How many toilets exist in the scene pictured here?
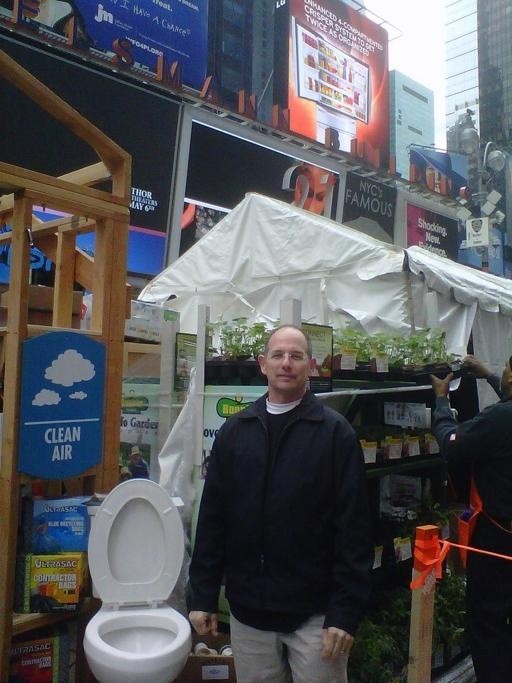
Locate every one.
[84,477,194,682]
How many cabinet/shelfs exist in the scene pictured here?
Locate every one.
[2,52,133,680]
[79,194,480,680]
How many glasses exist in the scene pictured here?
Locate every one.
[266,350,309,361]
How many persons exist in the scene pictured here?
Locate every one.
[188,324,376,682]
[118,445,150,484]
[292,162,336,219]
[424,345,511,682]
